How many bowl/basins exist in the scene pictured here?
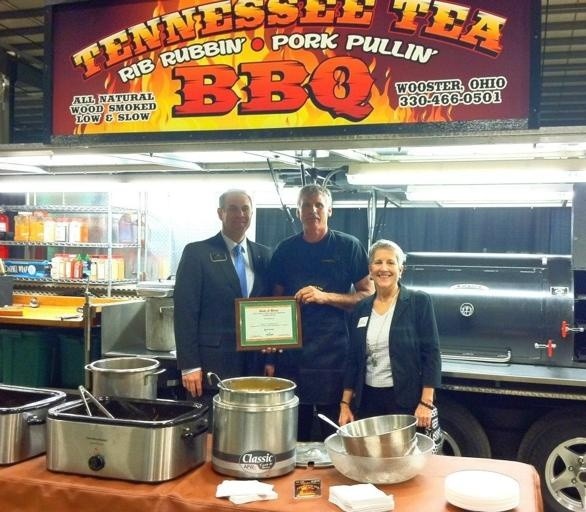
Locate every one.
[336,414,418,457]
[323,433,435,485]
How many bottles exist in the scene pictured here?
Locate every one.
[49,253,124,280]
[0,209,144,244]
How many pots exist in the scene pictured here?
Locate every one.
[83,358,166,401]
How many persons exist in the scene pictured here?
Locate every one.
[261,185,375,443]
[173,191,273,433]
[339,239,442,434]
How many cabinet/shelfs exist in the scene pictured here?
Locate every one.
[0,205,149,289]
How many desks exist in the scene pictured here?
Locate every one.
[0,434,542,512]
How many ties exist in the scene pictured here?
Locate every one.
[235,246,248,298]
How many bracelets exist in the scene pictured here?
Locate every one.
[339,399,351,406]
[419,400,434,412]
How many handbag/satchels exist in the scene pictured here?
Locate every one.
[421,404,444,454]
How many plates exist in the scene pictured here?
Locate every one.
[442,469,520,511]
